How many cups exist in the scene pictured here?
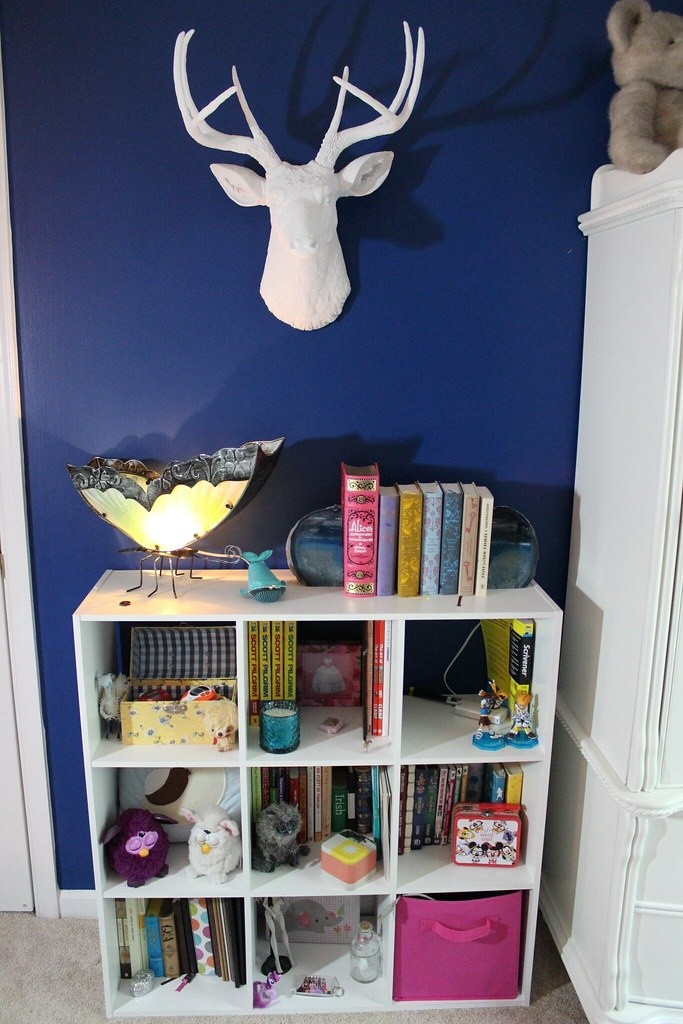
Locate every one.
[258,699,300,754]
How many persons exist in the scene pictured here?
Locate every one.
[262,896,295,975]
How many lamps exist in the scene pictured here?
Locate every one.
[64,436,288,601]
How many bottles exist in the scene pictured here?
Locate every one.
[349,921,380,984]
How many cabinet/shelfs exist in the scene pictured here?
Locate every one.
[71,566,563,1017]
[537,148,683,1024]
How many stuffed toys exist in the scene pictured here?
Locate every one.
[255,801,311,872]
[97,669,132,744]
[182,806,243,886]
[101,806,179,886]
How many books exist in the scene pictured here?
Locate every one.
[477,618,534,718]
[252,768,381,861]
[338,462,488,598]
[112,896,247,990]
[400,764,524,854]
[363,620,389,740]
[248,621,298,728]
